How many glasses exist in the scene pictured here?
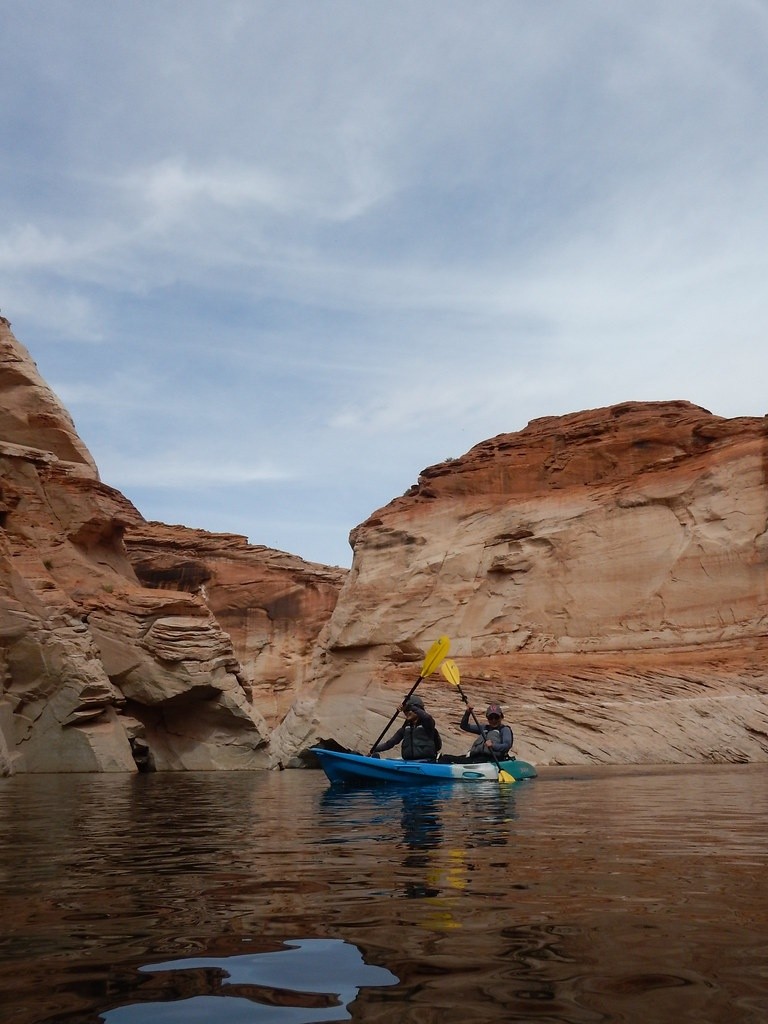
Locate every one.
[489,716,499,720]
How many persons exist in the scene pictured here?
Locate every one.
[441,701,514,764]
[366,696,442,763]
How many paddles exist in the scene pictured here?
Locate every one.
[367,635,450,756]
[440,659,517,782]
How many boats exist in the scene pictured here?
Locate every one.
[309,748,538,788]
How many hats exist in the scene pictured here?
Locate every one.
[405,695,426,711]
[486,705,502,718]
